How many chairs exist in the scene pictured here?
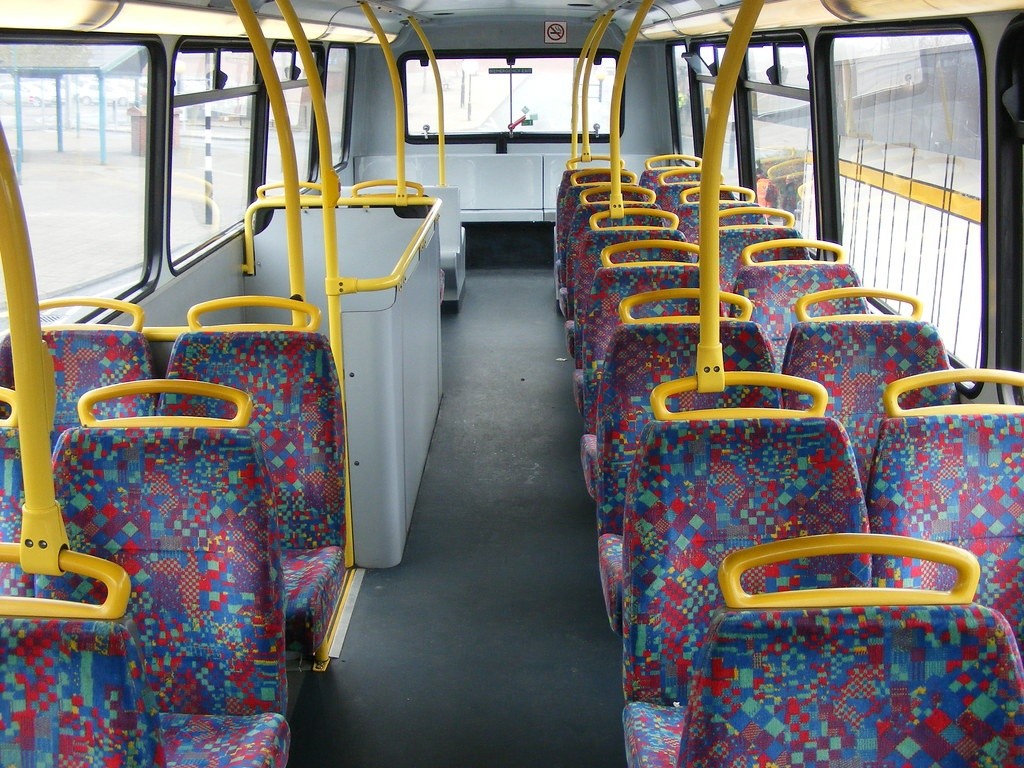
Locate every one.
[0,149,1022,764]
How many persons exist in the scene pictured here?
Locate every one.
[781,176,797,226]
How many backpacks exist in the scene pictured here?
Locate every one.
[765,182,777,202]
